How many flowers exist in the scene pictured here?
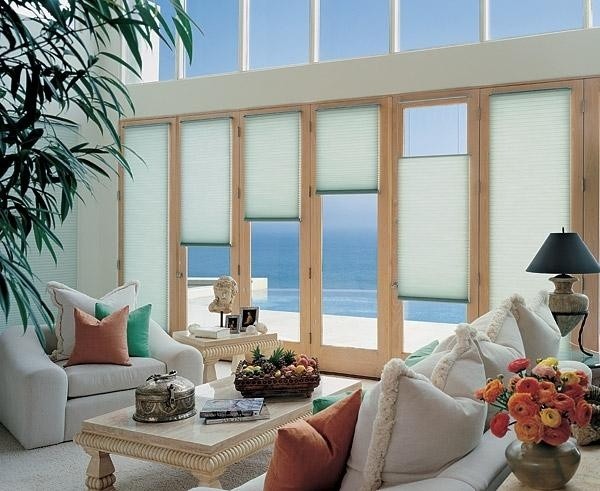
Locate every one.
[475,356,594,445]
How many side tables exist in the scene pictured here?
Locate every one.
[171,325,280,384]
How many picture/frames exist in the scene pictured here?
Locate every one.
[223,306,260,334]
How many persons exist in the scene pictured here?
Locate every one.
[228,318,236,330]
[242,311,253,327]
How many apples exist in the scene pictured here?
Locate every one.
[283,354,316,375]
[242,365,262,376]
[273,370,281,378]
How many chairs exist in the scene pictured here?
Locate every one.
[1,315,203,452]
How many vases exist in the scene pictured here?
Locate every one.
[502,431,582,490]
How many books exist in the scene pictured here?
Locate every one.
[204,403,271,425]
[199,396,265,418]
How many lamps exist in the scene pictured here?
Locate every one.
[521,229,599,358]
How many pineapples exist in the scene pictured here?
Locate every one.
[277,350,297,367]
[252,346,267,367]
[263,347,284,374]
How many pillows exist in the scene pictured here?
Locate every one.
[46,280,153,367]
